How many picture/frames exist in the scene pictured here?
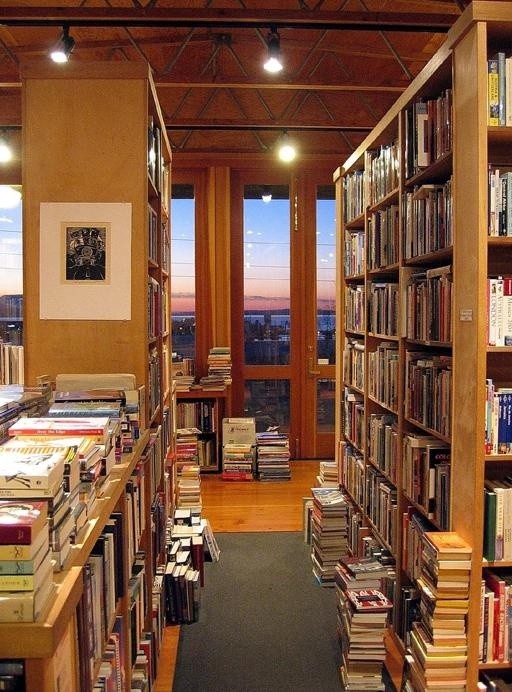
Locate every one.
[59,221,111,285]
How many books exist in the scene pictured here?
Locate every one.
[147,117,171,419]
[478,573,510,663]
[2,373,220,691]
[486,52,511,127]
[340,89,453,343]
[333,556,393,689]
[340,337,453,638]
[171,346,292,482]
[487,164,510,237]
[484,379,510,455]
[482,479,510,562]
[304,462,373,587]
[404,532,473,690]
[2,344,25,384]
[487,275,510,347]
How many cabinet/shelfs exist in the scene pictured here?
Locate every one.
[50,37,76,63]
[22,60,182,631]
[333,1,512,692]
[176,385,228,472]
[0,433,179,692]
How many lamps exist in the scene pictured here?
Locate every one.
[264,33,282,73]
